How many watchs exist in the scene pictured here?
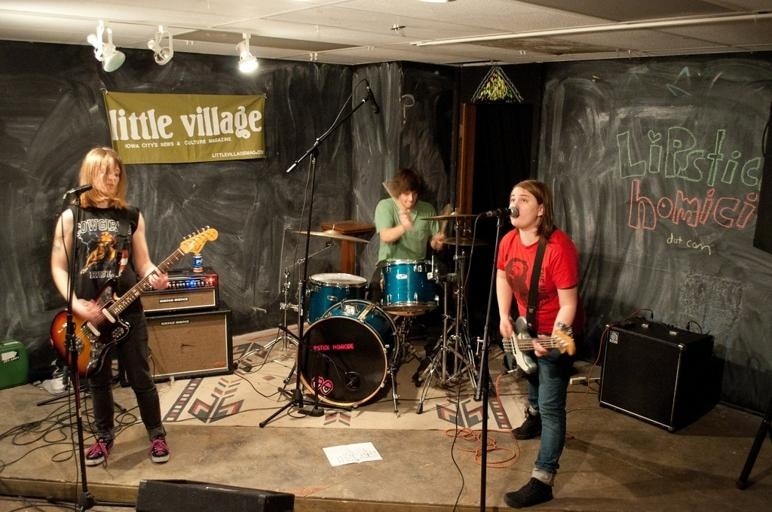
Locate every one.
[555,322,575,338]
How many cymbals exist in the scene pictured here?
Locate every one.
[419,208,476,220]
[291,225,372,244]
[444,237,487,247]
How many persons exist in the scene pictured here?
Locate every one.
[503,258,529,296]
[368,169,448,365]
[50,146,171,467]
[496,180,580,509]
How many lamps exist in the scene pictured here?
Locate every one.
[84,16,259,76]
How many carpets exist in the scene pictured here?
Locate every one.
[149,339,526,435]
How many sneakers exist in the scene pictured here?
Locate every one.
[149,435,170,462]
[512,407,543,440]
[84,436,114,466]
[504,477,554,509]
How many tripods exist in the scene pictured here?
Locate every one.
[414,222,496,414]
[233,243,335,373]
[258,96,366,429]
[47,194,136,512]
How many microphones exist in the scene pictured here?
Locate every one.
[487,208,519,217]
[366,83,380,114]
[69,185,92,195]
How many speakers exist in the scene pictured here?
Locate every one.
[600,317,713,432]
[118,300,234,388]
[137,479,294,512]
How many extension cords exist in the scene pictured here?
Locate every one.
[570,377,588,385]
[589,378,601,384]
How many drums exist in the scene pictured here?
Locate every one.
[298,300,399,407]
[379,258,439,315]
[306,273,369,326]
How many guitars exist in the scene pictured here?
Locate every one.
[51,225,219,377]
[500,317,575,379]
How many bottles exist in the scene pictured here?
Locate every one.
[191,253,204,273]
